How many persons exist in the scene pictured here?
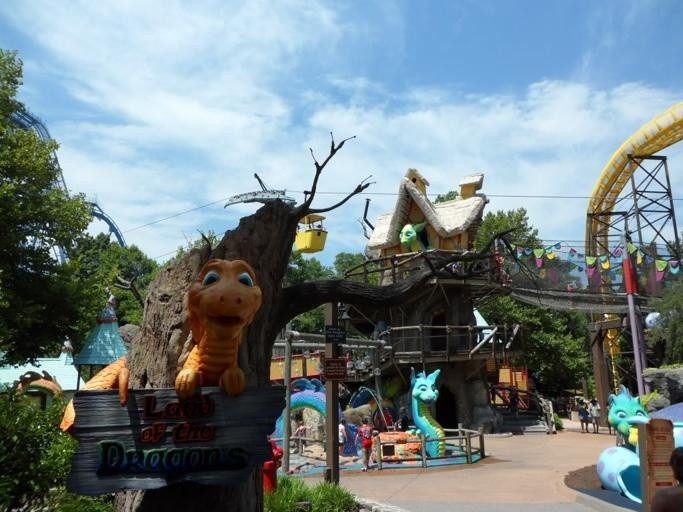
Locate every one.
[291,420,313,447]
[338,418,347,455]
[355,417,373,472]
[398,405,409,431]
[574,396,616,435]
